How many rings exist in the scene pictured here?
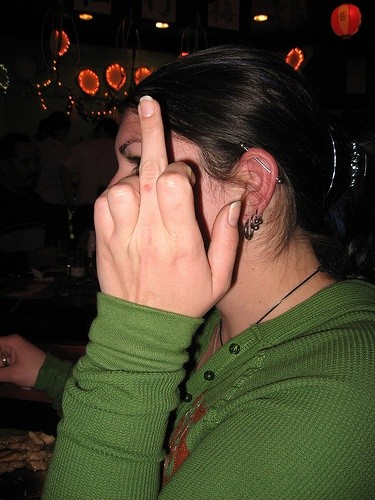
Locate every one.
[2,355,7,367]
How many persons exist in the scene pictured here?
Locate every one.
[1,133,63,278]
[0,45,375,500]
[63,119,118,249]
[34,109,75,239]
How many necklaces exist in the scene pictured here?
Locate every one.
[218,264,320,348]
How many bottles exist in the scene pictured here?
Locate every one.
[66,248,85,288]
